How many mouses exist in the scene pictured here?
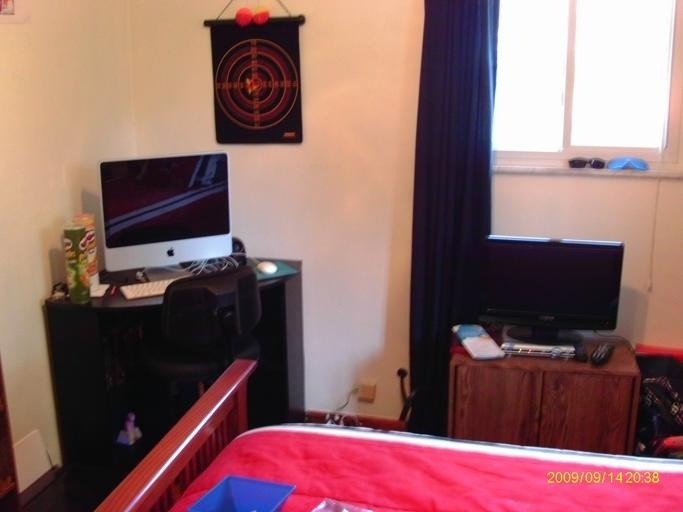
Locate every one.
[256,261,277,274]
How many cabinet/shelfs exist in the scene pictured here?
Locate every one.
[46,258,303,510]
[445,334,641,454]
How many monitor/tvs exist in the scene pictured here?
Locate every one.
[479,236,623,345]
[98,150,233,281]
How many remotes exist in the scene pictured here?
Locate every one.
[576,345,587,361]
[592,343,615,364]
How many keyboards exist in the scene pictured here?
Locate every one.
[120,278,181,300]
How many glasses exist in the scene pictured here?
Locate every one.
[567,157,605,169]
[606,156,649,171]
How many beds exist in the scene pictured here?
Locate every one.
[94,359,683,510]
